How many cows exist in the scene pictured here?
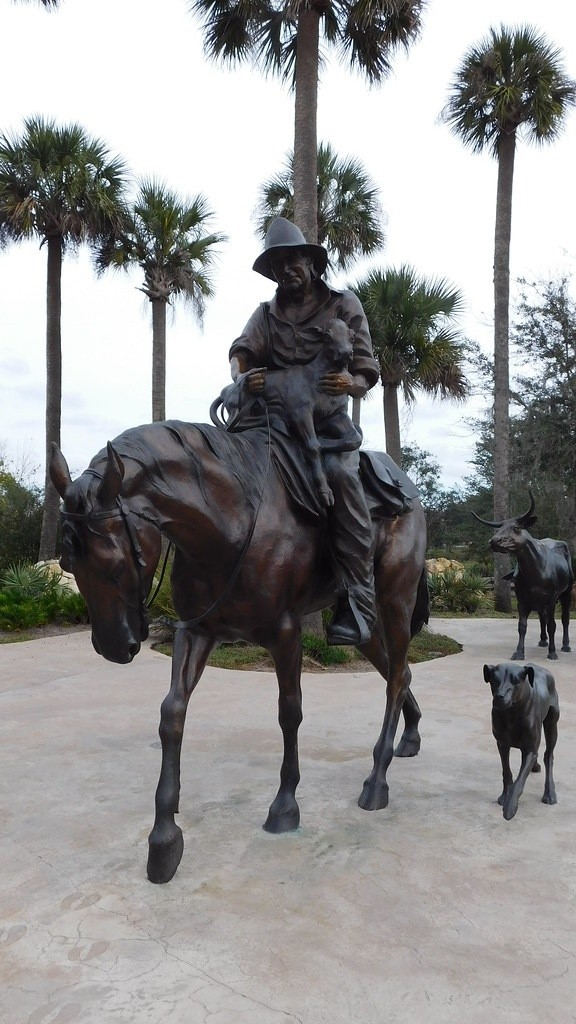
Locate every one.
[469,488,574,661]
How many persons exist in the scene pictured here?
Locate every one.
[227,215,380,646]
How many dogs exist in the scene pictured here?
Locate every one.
[483,661,558,820]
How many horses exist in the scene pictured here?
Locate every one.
[48,418,432,885]
